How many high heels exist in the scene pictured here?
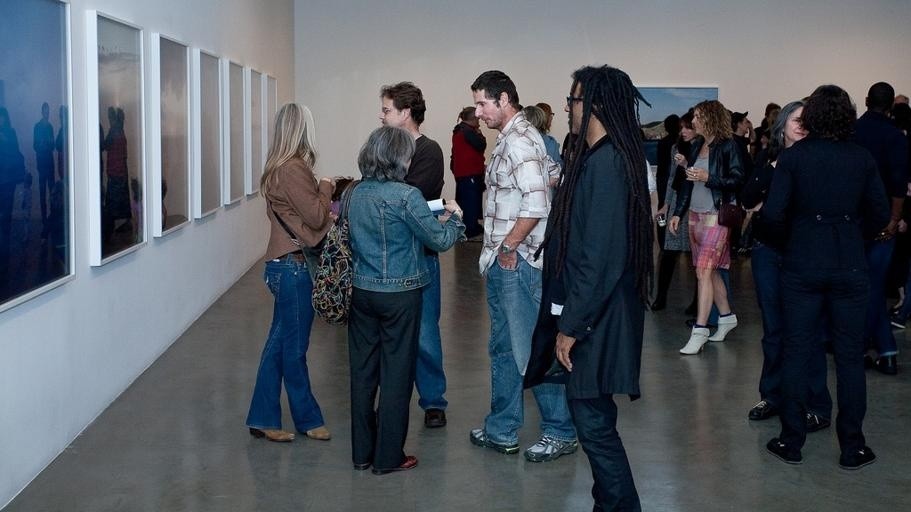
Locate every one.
[679,325,711,355]
[708,314,739,343]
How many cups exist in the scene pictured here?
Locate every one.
[689,167,698,170]
[657,215,667,226]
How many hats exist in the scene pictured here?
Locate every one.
[730,110,747,130]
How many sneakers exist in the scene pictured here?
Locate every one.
[748,400,777,420]
[839,448,874,470]
[807,413,831,432]
[766,438,802,462]
[523,435,577,463]
[466,234,484,243]
[874,353,898,375]
[891,306,908,330]
[471,429,520,454]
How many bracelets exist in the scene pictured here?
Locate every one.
[451,209,465,217]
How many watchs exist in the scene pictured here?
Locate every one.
[499,243,518,254]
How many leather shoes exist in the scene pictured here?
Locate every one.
[652,298,667,310]
[684,301,697,314]
[354,456,417,475]
[249,425,295,441]
[307,426,331,441]
[424,407,447,428]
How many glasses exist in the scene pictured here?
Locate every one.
[566,95,582,106]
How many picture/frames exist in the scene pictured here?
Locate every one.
[193,46,222,216]
[263,73,278,168]
[0,0,75,311]
[224,57,246,204]
[247,68,266,196]
[84,9,148,267]
[153,34,192,239]
[631,87,718,142]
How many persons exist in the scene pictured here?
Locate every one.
[469,70,579,462]
[645,83,910,470]
[533,64,655,512]
[1,78,168,266]
[378,81,448,429]
[340,127,467,473]
[451,104,574,242]
[331,176,354,217]
[246,105,336,443]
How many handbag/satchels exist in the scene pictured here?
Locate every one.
[299,245,321,281]
[717,202,745,230]
[312,219,354,328]
